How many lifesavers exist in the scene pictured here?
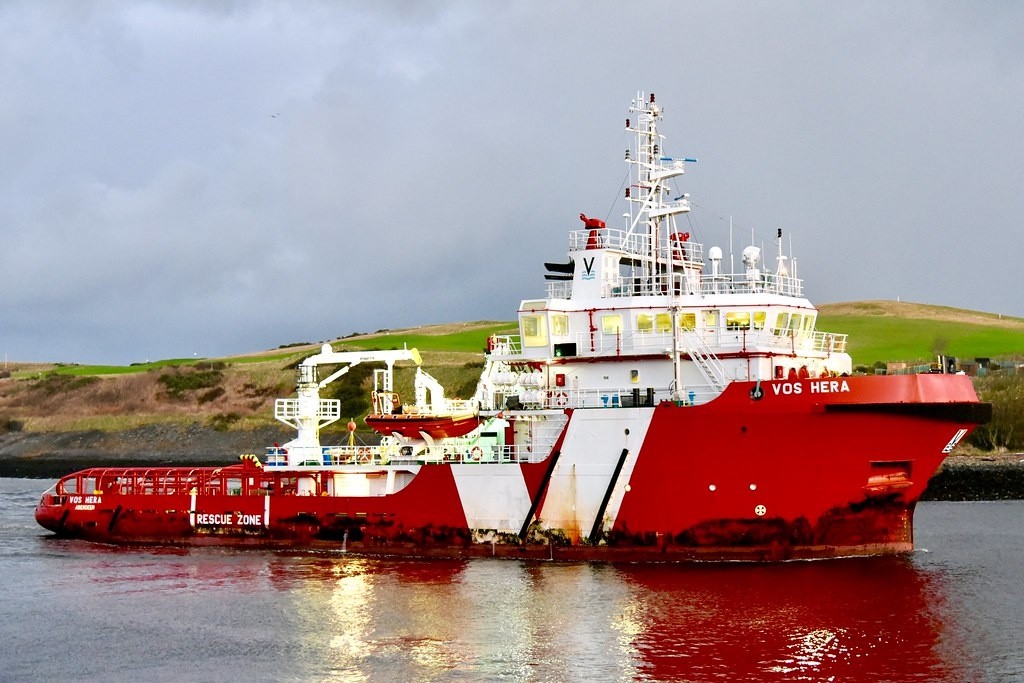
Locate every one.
[470,447,484,461]
[556,393,569,406]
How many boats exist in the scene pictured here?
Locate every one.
[34,93,992,559]
